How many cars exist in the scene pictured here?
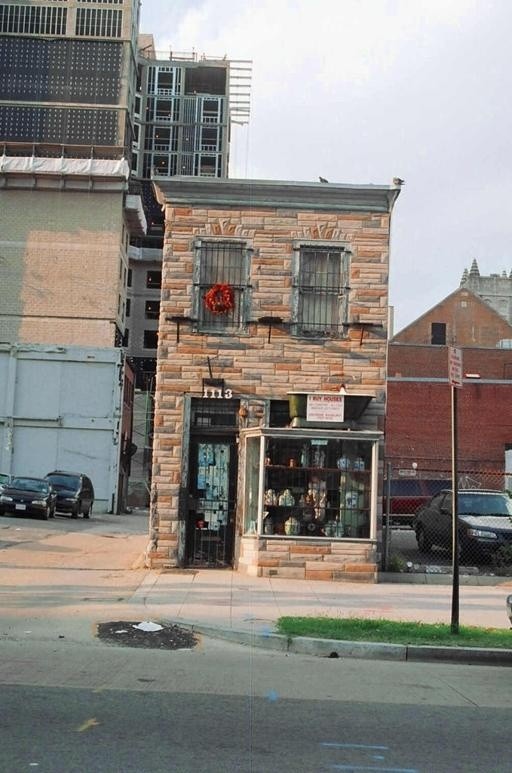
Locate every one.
[0,471,57,518]
[415,487,512,564]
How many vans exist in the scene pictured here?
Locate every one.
[384,477,453,526]
[43,469,95,520]
